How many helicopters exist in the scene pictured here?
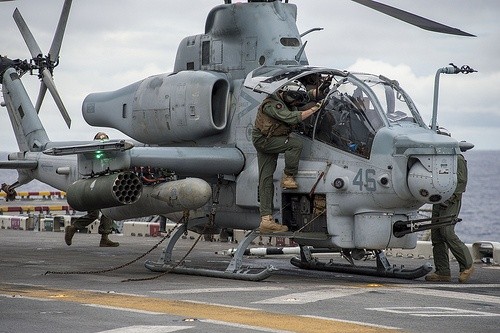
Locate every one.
[0,0,479,280]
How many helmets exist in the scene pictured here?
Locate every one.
[282,80,307,92]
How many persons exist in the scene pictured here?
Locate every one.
[425,154,473,283]
[65,209,119,247]
[252,82,324,234]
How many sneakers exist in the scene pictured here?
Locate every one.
[425,272,451,282]
[65,226,74,246]
[281,176,298,187]
[458,268,473,281]
[260,220,288,232]
[100,239,119,247]
